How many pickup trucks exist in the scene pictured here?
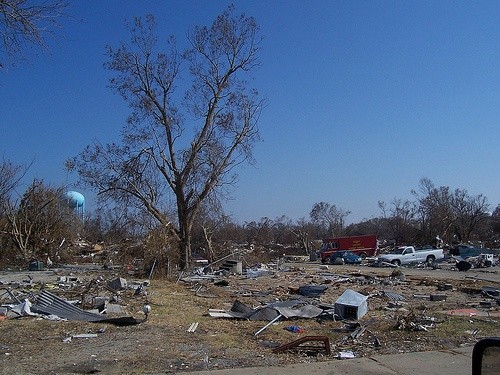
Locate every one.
[378,246,443,267]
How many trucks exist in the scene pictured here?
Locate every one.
[321,235,376,263]
